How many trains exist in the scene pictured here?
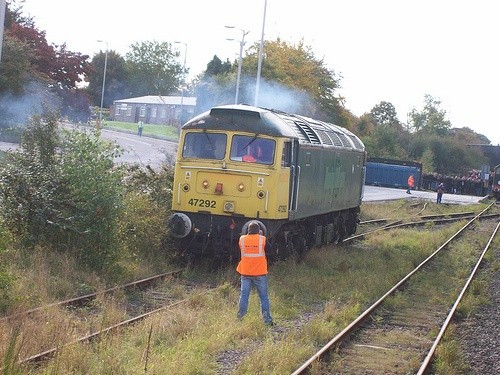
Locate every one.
[492,163,500,203]
[165,104,366,264]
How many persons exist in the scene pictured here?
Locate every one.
[406,169,495,204]
[137,119,144,137]
[236,221,277,326]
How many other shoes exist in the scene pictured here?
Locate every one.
[406,191,412,194]
[269,322,276,327]
